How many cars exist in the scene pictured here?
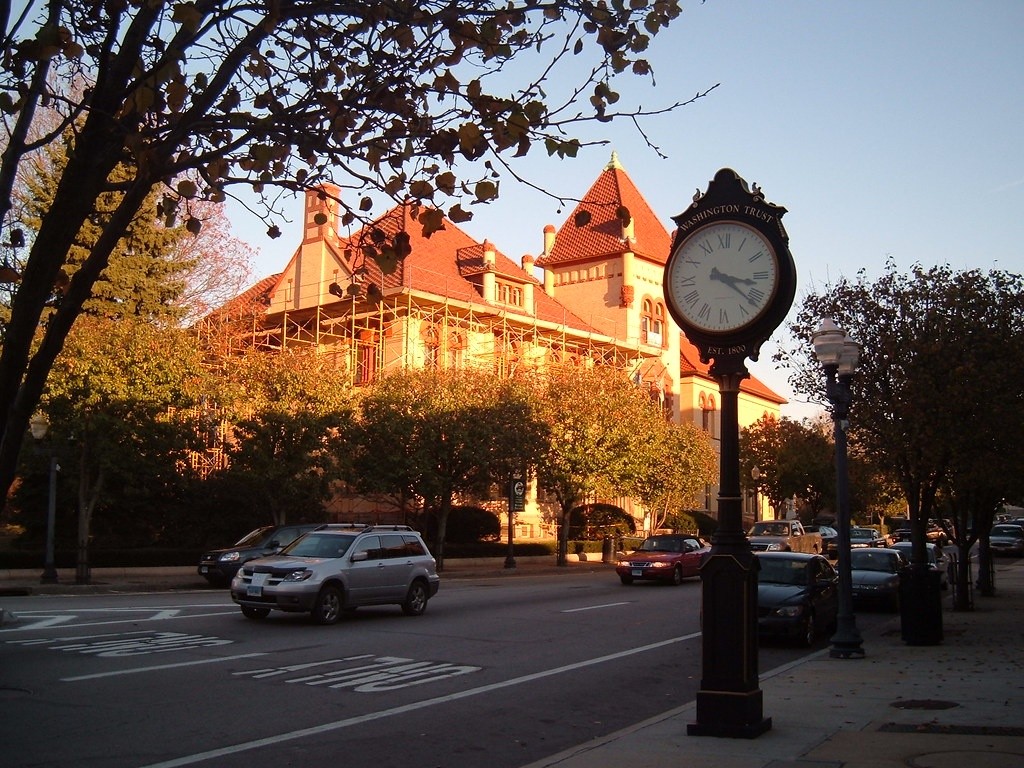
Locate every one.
[891,542,950,590]
[615,534,712,586]
[198,524,323,588]
[933,519,956,540]
[827,528,889,560]
[834,548,910,612]
[989,525,1024,556]
[893,519,947,545]
[699,552,839,648]
[792,525,838,554]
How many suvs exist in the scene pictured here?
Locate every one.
[231,523,440,625]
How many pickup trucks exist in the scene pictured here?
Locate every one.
[745,520,822,555]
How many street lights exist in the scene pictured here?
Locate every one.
[29,408,59,583]
[811,315,865,659]
[751,465,760,522]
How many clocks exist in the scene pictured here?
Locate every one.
[667,219,780,335]
